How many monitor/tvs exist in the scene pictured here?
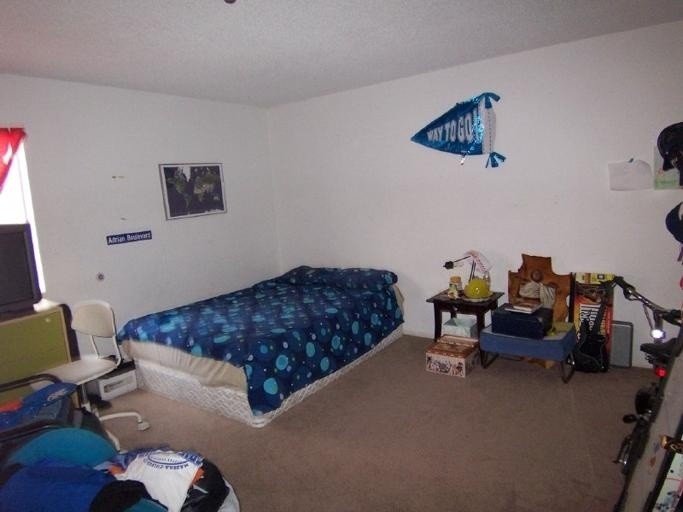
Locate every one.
[0,223,42,315]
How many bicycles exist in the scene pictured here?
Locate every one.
[611,274,683,511]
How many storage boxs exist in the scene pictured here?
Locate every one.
[85,361,138,399]
[443,317,477,337]
[492,302,554,340]
[425,335,480,378]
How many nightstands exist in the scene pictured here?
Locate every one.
[426,289,504,369]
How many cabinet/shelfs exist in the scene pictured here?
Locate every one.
[0,297,81,411]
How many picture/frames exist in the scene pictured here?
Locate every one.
[159,162,229,221]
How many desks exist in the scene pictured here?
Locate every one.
[479,324,577,384]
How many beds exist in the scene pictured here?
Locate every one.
[116,264,404,432]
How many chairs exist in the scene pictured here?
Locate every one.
[30,300,152,455]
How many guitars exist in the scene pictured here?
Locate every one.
[574,285,614,374]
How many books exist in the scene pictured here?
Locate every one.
[505,299,543,315]
